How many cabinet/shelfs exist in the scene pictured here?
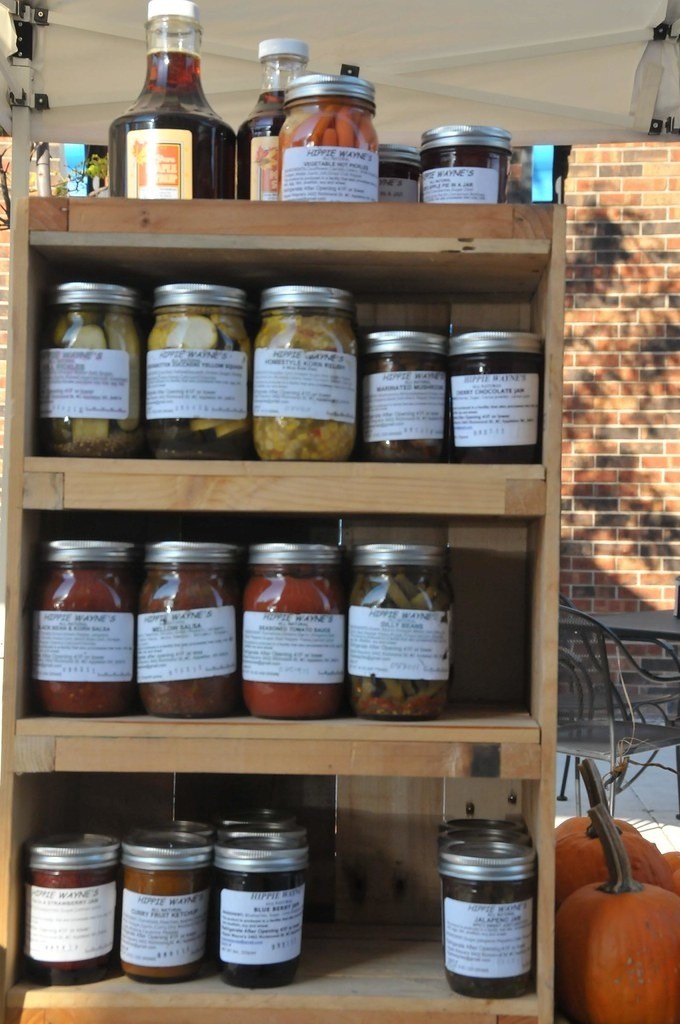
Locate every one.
[0,190,566,1024]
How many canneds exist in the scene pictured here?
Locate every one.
[437,818,536,1000]
[37,282,544,468]
[30,539,450,721]
[22,810,311,989]
[276,72,513,204]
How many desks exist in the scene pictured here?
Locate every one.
[560,609,680,671]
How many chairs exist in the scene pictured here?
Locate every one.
[557,603,680,818]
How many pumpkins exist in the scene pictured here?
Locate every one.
[553,758,680,1024]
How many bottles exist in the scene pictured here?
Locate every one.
[237,37,310,199]
[108,0,237,198]
